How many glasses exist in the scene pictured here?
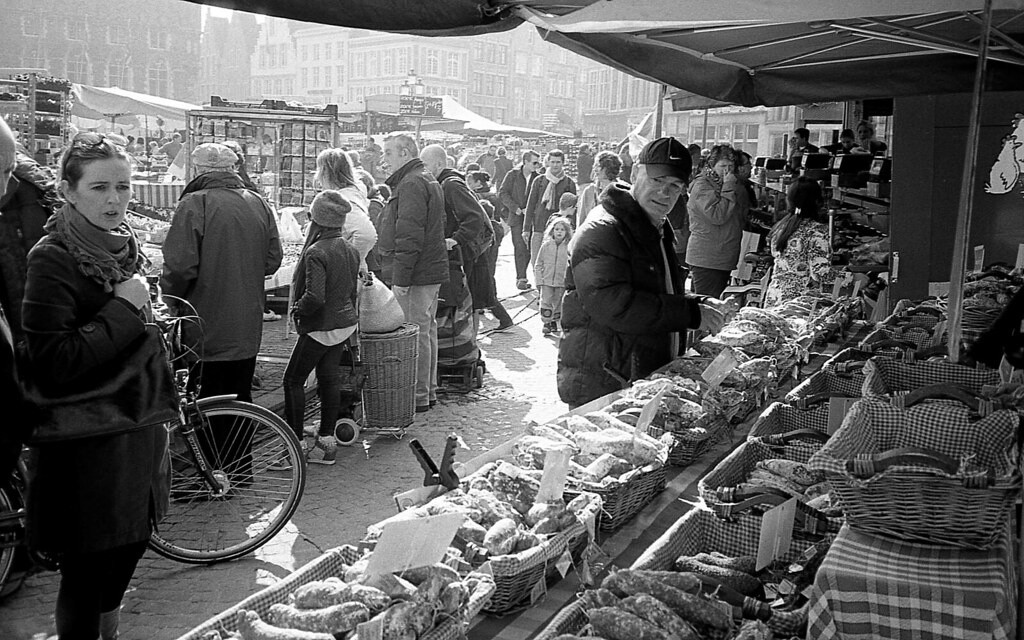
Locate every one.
[64,133,130,179]
[526,160,539,165]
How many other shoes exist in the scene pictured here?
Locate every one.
[306,440,337,465]
[543,324,552,337]
[516,280,531,289]
[550,321,558,333]
[262,310,281,320]
[493,319,514,333]
[173,483,235,501]
[267,452,294,471]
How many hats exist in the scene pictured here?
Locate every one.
[190,143,239,168]
[310,189,350,228]
[639,138,691,189]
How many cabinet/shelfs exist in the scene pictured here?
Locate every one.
[0,73,72,173]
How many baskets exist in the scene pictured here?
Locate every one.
[861,355,1024,420]
[809,398,1024,552]
[958,304,1004,332]
[181,296,863,640]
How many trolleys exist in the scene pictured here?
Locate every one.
[434,245,486,391]
[333,271,415,447]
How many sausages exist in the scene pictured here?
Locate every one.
[548,550,780,640]
[961,276,1009,309]
[209,413,659,640]
[738,458,837,511]
[605,294,840,431]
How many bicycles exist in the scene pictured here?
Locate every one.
[0,272,308,603]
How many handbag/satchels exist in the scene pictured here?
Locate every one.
[16,237,183,442]
[356,270,403,334]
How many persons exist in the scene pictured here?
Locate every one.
[0,119,888,577]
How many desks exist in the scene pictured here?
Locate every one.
[807,502,1021,640]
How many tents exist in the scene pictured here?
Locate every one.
[71,85,199,157]
